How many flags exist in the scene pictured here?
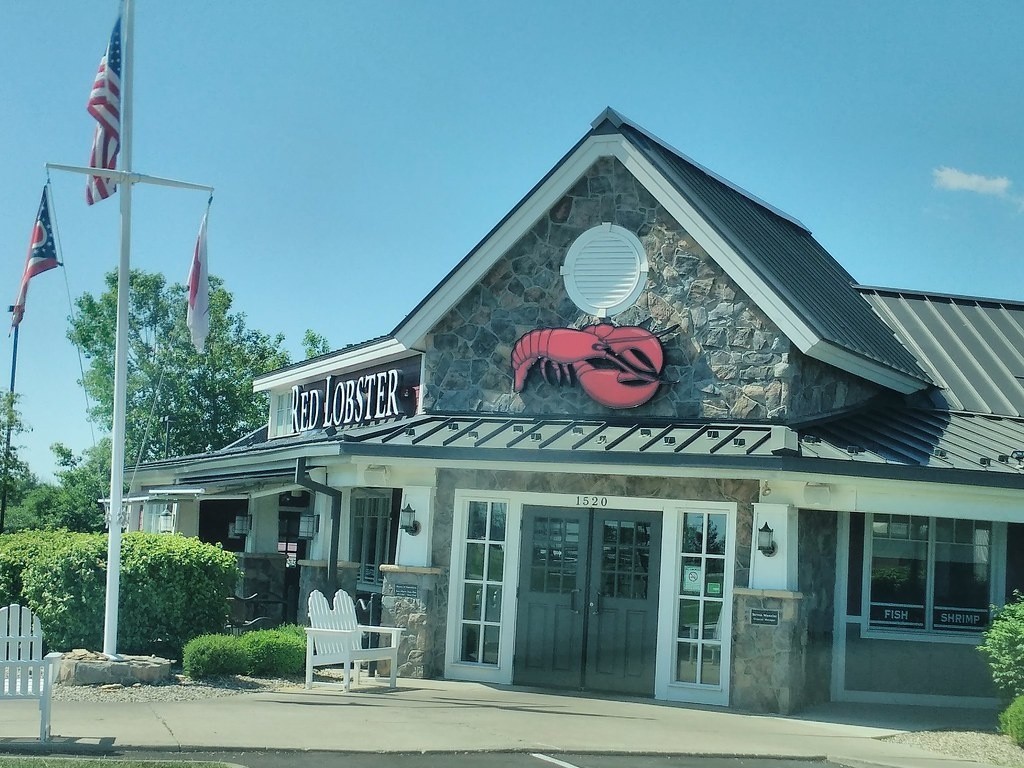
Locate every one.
[84,16,123,207]
[10,184,60,328]
[186,200,211,354]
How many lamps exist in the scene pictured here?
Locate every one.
[298,506,320,540]
[159,504,175,534]
[234,508,252,537]
[228,516,244,539]
[399,503,421,536]
[757,521,778,557]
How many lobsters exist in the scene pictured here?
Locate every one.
[510,317,681,409]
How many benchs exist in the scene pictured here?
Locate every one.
[303,588,408,694]
[1,604,63,742]
